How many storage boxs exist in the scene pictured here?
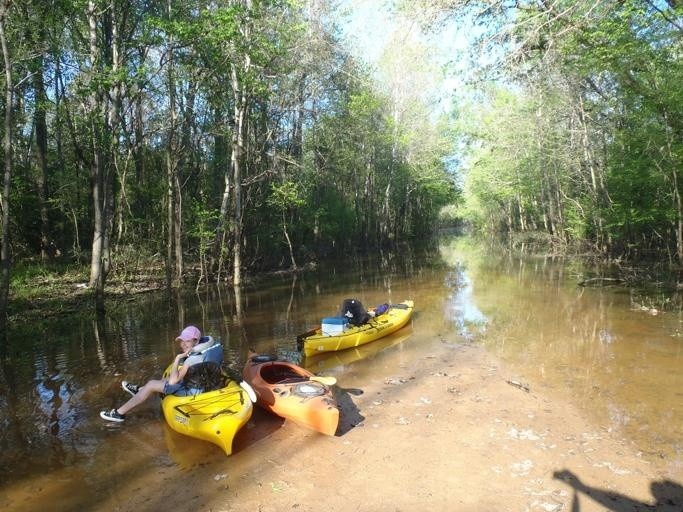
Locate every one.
[321,317,348,337]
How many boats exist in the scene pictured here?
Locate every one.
[244,349,341,435]
[296,300,414,357]
[162,361,254,456]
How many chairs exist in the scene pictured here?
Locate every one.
[184,361,222,394]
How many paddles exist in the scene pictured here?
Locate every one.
[275,372,336,386]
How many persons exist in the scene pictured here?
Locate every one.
[99,325,223,423]
[338,299,373,327]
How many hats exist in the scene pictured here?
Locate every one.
[175,325,202,345]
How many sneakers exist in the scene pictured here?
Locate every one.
[99,408,126,423]
[122,380,140,396]
